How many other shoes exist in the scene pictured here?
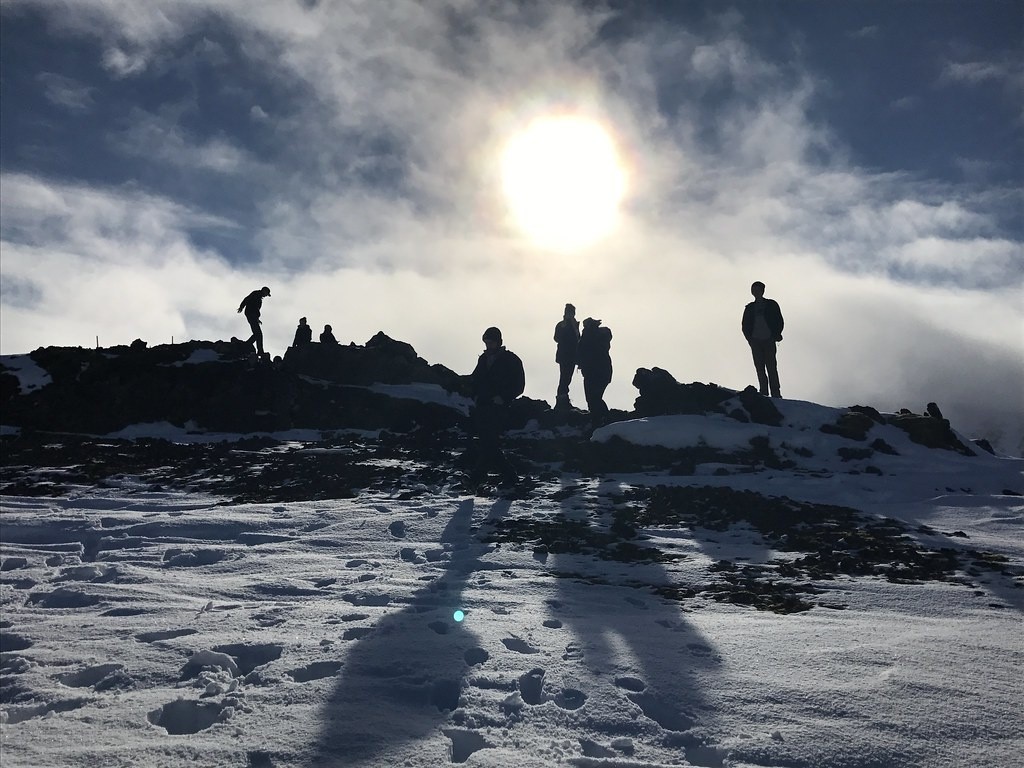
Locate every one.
[257,351,267,355]
[554,396,570,417]
[502,465,518,488]
[461,474,475,494]
[585,422,604,440]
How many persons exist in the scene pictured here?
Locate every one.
[742,281,784,398]
[292,317,311,349]
[576,318,613,412]
[554,304,580,411]
[443,327,526,489]
[320,325,338,345]
[239,287,271,353]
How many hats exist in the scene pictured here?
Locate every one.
[483,327,502,342]
[300,317,306,323]
[263,287,271,297]
[751,282,765,294]
